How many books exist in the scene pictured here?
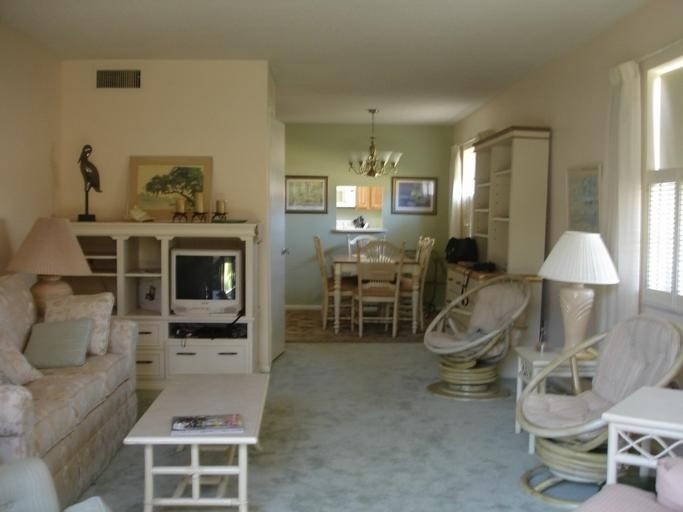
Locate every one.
[170,414,245,436]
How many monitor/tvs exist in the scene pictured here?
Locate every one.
[170,248,243,314]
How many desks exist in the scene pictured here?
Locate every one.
[601,385,683,485]
[513,345,601,455]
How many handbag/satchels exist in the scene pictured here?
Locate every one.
[472,261,496,273]
[445,237,479,264]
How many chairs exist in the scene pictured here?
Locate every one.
[512,314,683,510]
[424,274,531,402]
[314,234,435,338]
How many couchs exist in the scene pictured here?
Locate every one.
[0,273,139,512]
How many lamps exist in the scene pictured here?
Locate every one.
[349,108,403,177]
[5,216,94,323]
[538,231,621,363]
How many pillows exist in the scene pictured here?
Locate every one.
[1,292,114,387]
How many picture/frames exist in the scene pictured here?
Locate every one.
[391,176,438,216]
[285,175,328,214]
[564,163,602,231]
[129,156,213,222]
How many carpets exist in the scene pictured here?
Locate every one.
[285,310,445,343]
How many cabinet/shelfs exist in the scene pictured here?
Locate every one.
[356,185,383,210]
[62,223,258,391]
[444,127,550,379]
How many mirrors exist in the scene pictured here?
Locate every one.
[336,185,384,229]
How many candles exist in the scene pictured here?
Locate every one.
[175,191,226,213]
